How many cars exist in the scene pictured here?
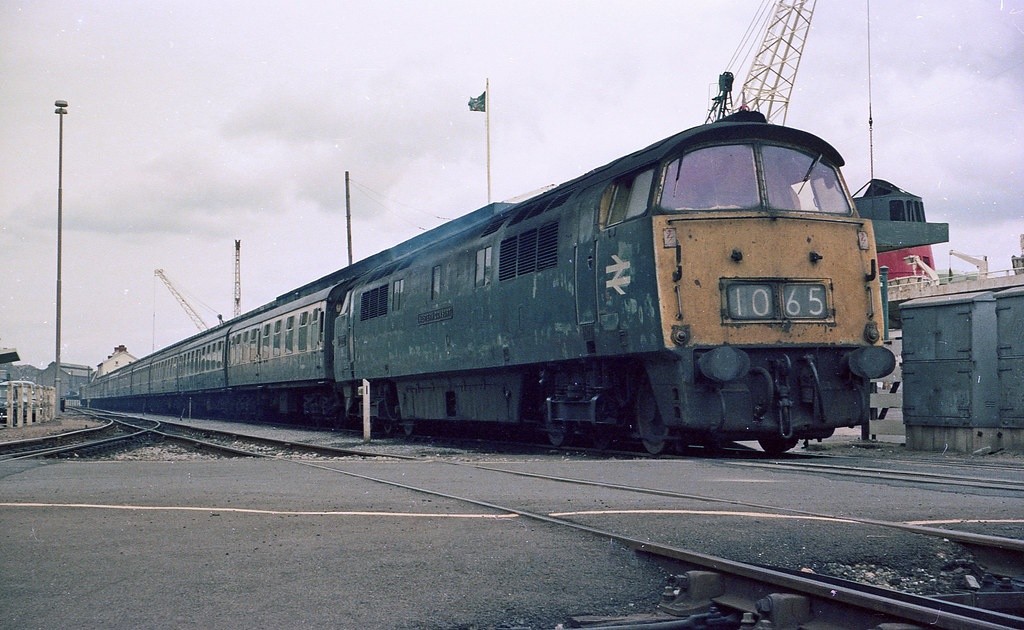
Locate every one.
[0,380,54,424]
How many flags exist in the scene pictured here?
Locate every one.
[468,91,486,112]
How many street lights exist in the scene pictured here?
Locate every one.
[54,100,68,422]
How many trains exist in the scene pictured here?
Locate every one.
[74,108,896,458]
